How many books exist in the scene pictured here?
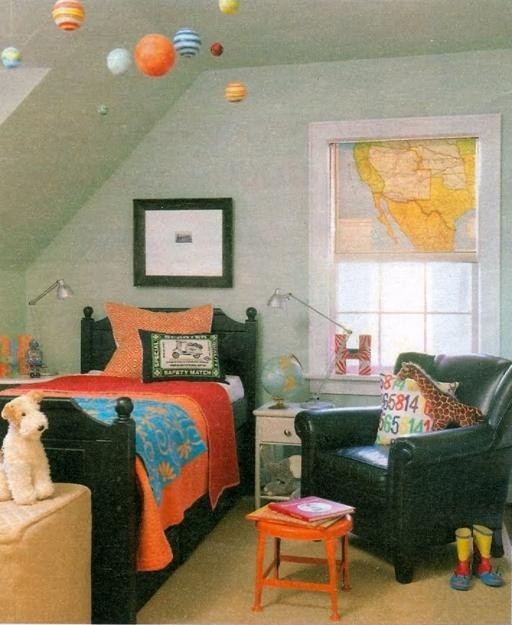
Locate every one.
[245,492,360,533]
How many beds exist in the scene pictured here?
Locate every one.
[1,304,262,624]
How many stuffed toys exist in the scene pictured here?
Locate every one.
[1,390,57,506]
[255,453,305,500]
[397,358,485,430]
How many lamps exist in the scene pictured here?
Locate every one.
[28,277,76,375]
[265,288,352,410]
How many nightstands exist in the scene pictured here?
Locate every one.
[253,402,331,509]
[1,376,67,387]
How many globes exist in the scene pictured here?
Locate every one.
[259,353,305,410]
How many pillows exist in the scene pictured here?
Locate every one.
[102,299,216,382]
[373,370,459,448]
[137,326,231,386]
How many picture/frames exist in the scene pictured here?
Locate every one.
[131,196,236,294]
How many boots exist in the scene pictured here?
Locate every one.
[450,526,474,593]
[472,523,504,588]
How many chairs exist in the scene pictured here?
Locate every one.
[293,351,511,585]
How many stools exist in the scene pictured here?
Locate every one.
[253,500,352,621]
[2,479,96,625]
